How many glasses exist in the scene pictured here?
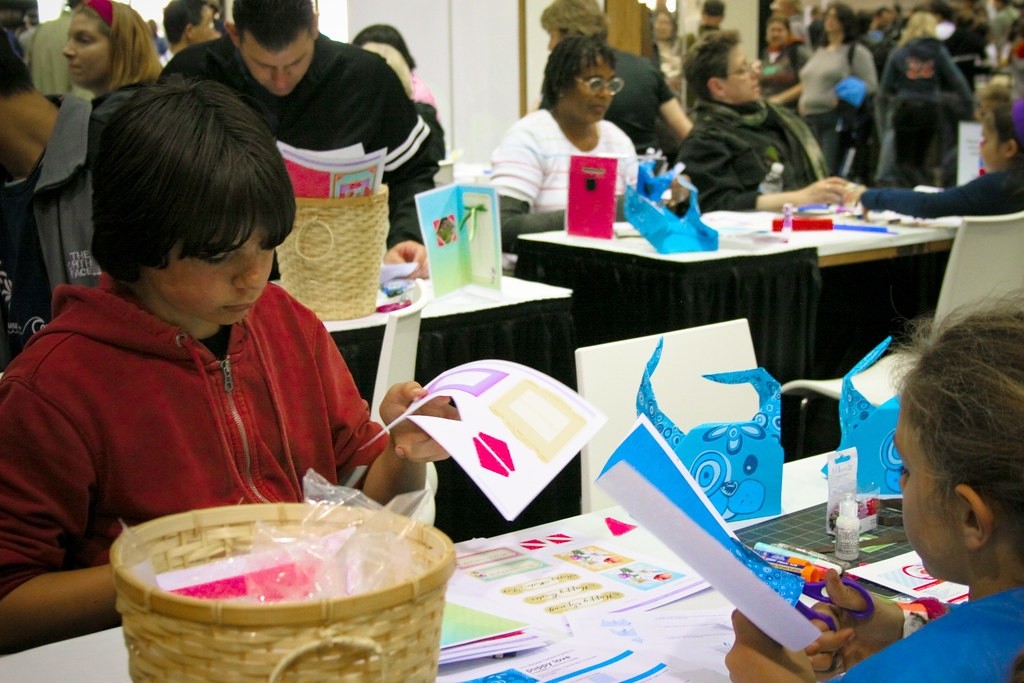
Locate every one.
[722,58,753,79]
[577,74,626,96]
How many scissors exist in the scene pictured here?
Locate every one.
[792,568,875,672]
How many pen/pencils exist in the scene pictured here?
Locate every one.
[840,146,857,180]
[835,222,888,233]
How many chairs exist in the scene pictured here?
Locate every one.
[931,211,1024,339]
[341,294,440,530]
[433,151,456,188]
[571,317,760,515]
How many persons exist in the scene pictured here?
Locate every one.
[0,0,446,403]
[487,0,1024,285]
[726,285,1024,683]
[0,68,461,659]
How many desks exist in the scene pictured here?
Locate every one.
[267,262,585,545]
[512,222,822,463]
[752,209,959,270]
[0,451,841,683]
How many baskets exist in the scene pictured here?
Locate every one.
[108,503,456,682]
[277,184,390,320]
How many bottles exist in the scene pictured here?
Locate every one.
[835,501,860,561]
[758,162,784,196]
